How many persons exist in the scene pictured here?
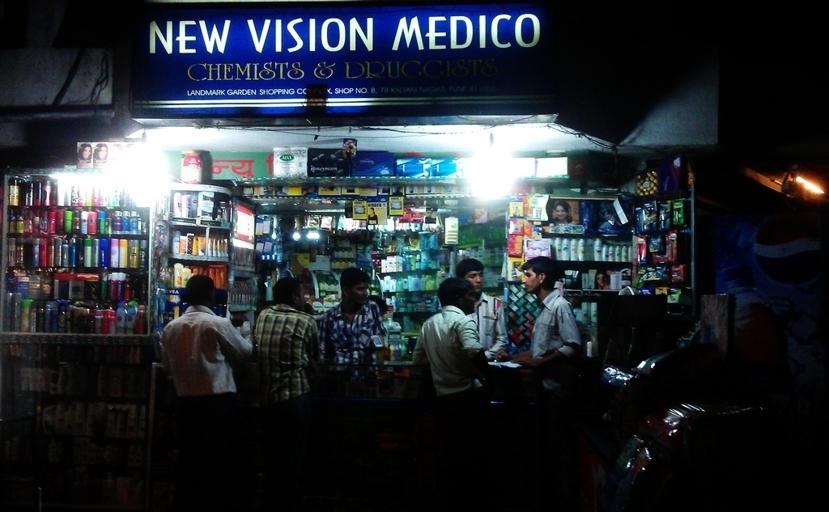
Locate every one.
[592,270,609,289]
[410,275,495,510]
[76,143,93,164]
[310,266,387,370]
[93,143,109,163]
[161,273,254,512]
[425,209,435,224]
[367,206,379,226]
[248,276,321,512]
[455,257,510,361]
[514,254,586,511]
[551,199,575,224]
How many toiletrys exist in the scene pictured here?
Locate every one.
[4,173,634,510]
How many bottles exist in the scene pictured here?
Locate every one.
[389,342,407,361]
[385,295,396,311]
[4,339,145,509]
[4,237,147,299]
[582,302,598,321]
[7,176,145,234]
[4,293,145,335]
[405,254,421,271]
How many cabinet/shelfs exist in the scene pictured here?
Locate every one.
[233,361,425,399]
[544,196,630,296]
[231,198,259,312]
[302,268,381,310]
[167,183,230,327]
[456,221,505,292]
[0,204,158,512]
[371,248,440,316]
